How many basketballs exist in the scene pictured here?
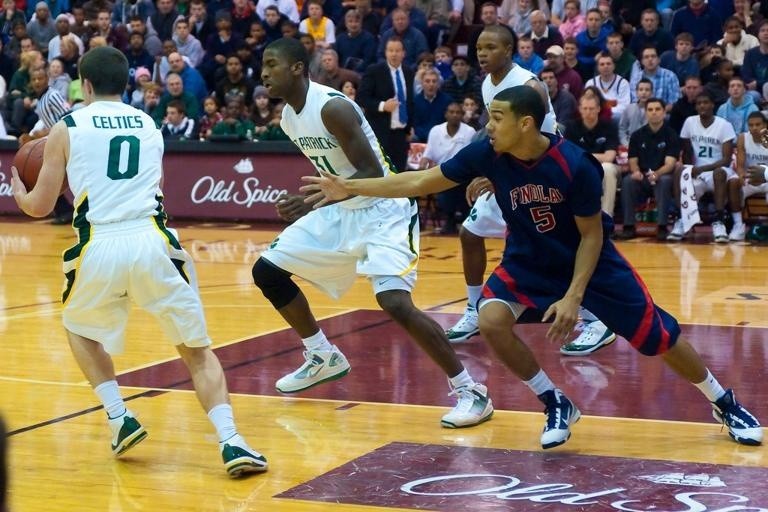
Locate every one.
[13,135,69,198]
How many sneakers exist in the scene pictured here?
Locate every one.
[106,408,149,458]
[711,389,764,446]
[535,388,581,449]
[609,221,765,244]
[439,224,457,234]
[559,320,617,356]
[445,303,480,342]
[441,375,493,427]
[219,432,267,480]
[276,345,350,394]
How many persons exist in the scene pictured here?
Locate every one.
[1,1,766,170]
[250,39,495,428]
[443,23,617,357]
[562,94,619,218]
[666,93,735,244]
[13,66,74,229]
[420,105,478,235]
[615,98,680,241]
[729,111,768,241]
[11,45,267,477]
[299,86,763,449]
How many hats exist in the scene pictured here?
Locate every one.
[546,45,564,56]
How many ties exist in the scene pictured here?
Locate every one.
[395,70,409,124]
